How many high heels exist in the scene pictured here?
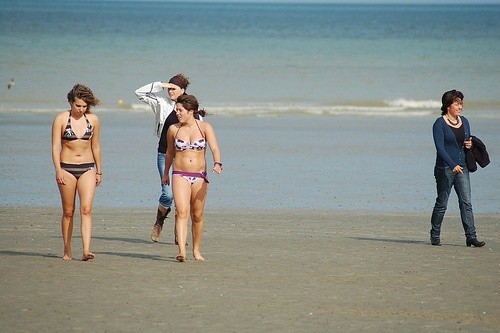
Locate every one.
[466,237,485,247]
[431,230,440,245]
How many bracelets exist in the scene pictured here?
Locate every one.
[96,172,102,175]
[214,162,222,170]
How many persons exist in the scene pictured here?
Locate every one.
[52,84,103,261]
[430,89,486,248]
[162,95,222,262]
[135,75,203,246]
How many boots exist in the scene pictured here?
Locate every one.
[151,207,171,241]
[175,215,188,246]
